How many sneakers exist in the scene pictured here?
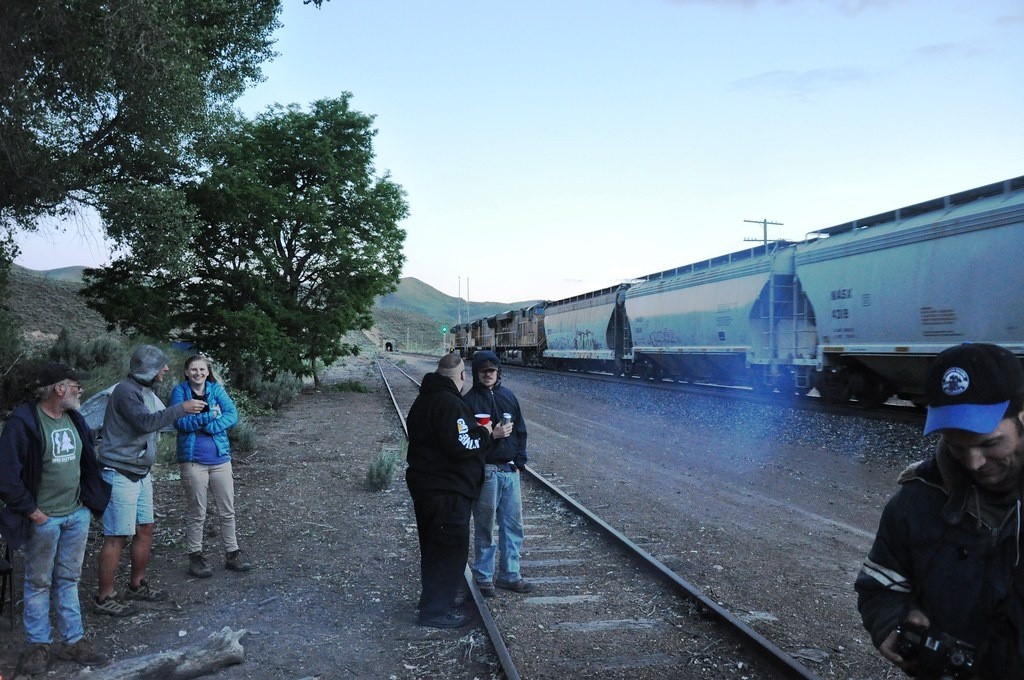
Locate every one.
[22,643,51,675]
[189,551,212,577]
[224,549,252,571]
[58,638,108,665]
[93,591,140,616]
[125,579,169,602]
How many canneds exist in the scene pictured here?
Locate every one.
[502,413,511,426]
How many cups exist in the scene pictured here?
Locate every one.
[475,414,490,425]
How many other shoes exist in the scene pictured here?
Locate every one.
[477,580,495,597]
[496,577,536,592]
[422,606,474,628]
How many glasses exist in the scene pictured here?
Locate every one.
[53,382,81,392]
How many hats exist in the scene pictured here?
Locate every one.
[478,361,499,372]
[921,342,1023,435]
[35,363,92,387]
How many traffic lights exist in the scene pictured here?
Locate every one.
[442,327,447,333]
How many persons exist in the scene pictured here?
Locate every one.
[0,363,113,674]
[170,355,251,578]
[405,353,514,628]
[93,344,208,617]
[462,350,533,597]
[856,343,1024,680]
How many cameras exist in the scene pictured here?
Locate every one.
[894,623,975,679]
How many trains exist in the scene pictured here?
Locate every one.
[450,175,1024,408]
